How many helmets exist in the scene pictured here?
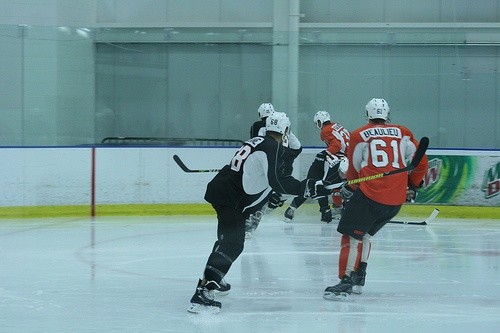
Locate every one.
[258,103,275,118]
[365,98,390,120]
[313,110,331,130]
[265,111,291,137]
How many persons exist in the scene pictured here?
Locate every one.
[251,103,350,224]
[324,98,428,302]
[187,112,327,314]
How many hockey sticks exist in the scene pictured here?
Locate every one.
[323,136,429,190]
[173,155,222,172]
[331,207,440,225]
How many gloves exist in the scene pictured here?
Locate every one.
[303,177,334,198]
[405,174,424,204]
[268,191,285,209]
[339,184,355,211]
[319,208,333,223]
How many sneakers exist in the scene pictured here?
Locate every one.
[283,207,295,223]
[323,274,354,301]
[187,279,221,314]
[244,211,264,238]
[350,262,368,294]
[202,277,232,296]
